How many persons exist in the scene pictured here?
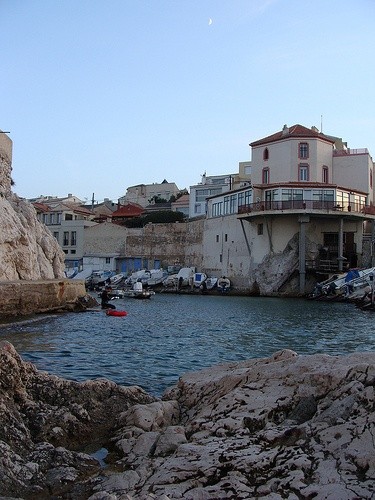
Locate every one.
[101,286,116,309]
[314,244,328,269]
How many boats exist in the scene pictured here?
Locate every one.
[315,265,375,309]
[125,279,155,299]
[97,285,124,300]
[67,268,231,294]
